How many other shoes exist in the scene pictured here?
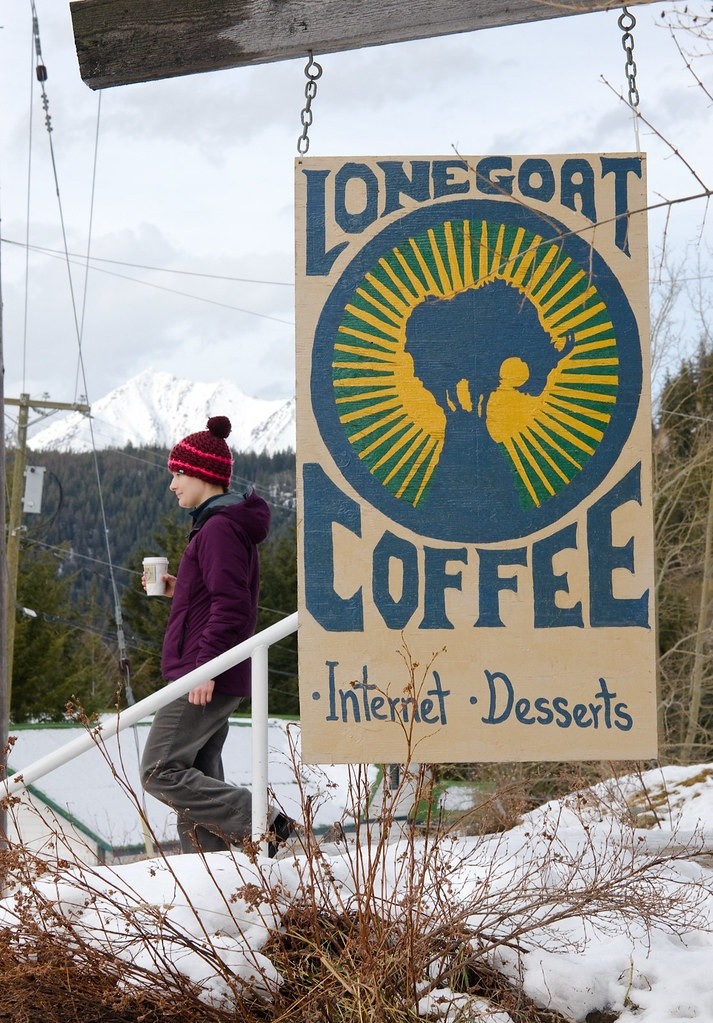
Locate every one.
[269,813,295,857]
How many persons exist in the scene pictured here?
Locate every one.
[139,416,299,858]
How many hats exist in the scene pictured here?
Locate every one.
[167,415,233,488]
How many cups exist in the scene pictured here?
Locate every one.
[141,557,169,597]
